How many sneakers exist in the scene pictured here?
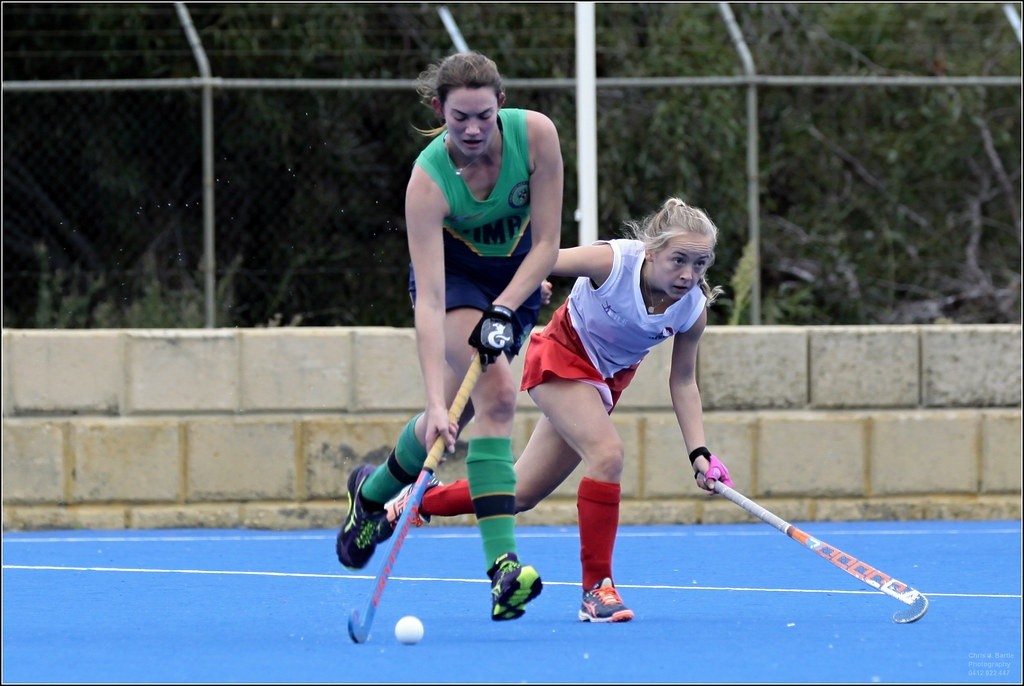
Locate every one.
[578,578,634,622]
[384,473,443,531]
[487,553,543,621]
[336,464,389,572]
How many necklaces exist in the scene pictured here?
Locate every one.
[644,272,666,313]
[445,139,486,176]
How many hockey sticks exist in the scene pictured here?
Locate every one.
[343,347,483,645]
[693,470,930,625]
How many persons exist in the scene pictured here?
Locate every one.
[376,193,733,623]
[335,51,564,620]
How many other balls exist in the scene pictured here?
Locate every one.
[394,616,424,646]
